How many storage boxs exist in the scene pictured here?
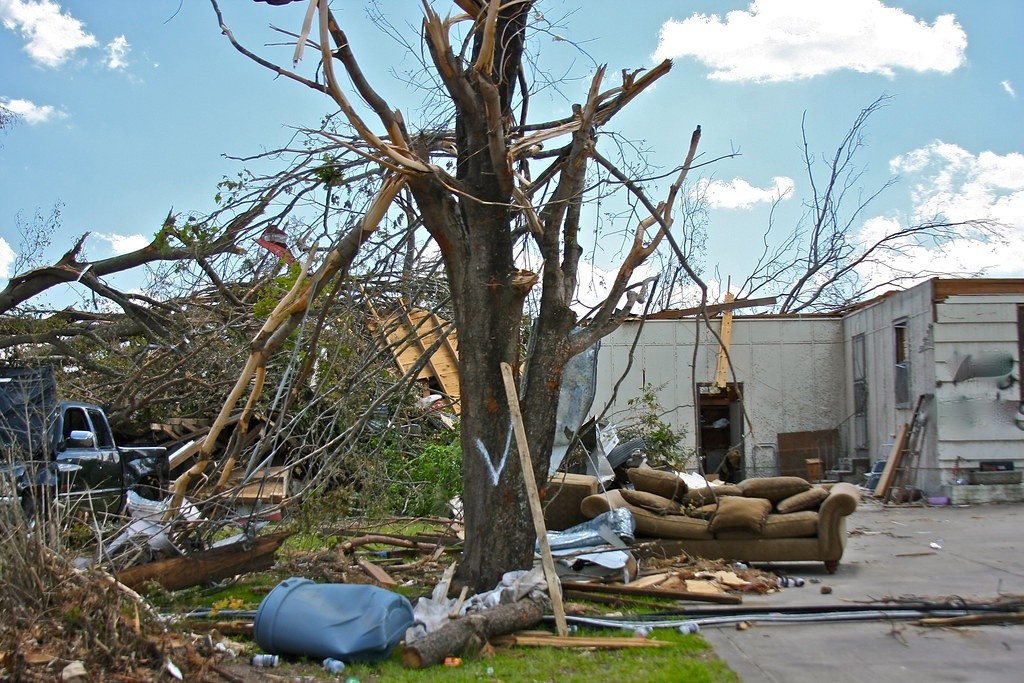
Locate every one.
[577,415,620,457]
[542,473,598,532]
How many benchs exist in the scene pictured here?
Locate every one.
[582,482,857,573]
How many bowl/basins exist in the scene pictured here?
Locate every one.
[957,479,970,485]
[927,497,950,505]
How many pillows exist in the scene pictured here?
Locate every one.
[627,467,829,535]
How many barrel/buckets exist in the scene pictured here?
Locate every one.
[253,575,414,665]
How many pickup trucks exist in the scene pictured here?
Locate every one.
[0,401,171,528]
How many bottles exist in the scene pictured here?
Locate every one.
[634,626,654,638]
[776,575,805,587]
[445,657,461,667]
[250,654,279,667]
[677,622,699,636]
[566,624,577,633]
[323,657,345,674]
[733,562,748,569]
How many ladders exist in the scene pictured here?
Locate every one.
[883,420,927,505]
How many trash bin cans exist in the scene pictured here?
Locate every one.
[253,576,414,661]
